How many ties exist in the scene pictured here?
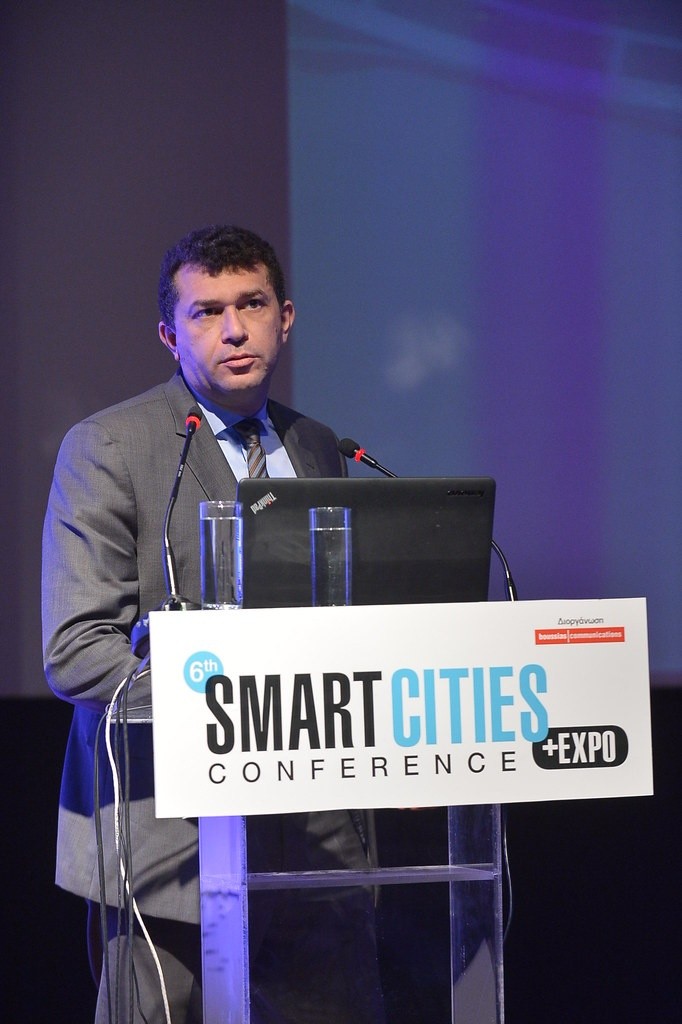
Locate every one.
[232,419,271,478]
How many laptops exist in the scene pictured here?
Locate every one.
[234,477,496,606]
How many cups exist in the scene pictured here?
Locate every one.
[199,501,243,610]
[308,507,353,606]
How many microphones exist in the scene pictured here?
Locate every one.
[337,439,517,601]
[131,404,201,662]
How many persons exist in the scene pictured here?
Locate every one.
[41,225,384,1024]
